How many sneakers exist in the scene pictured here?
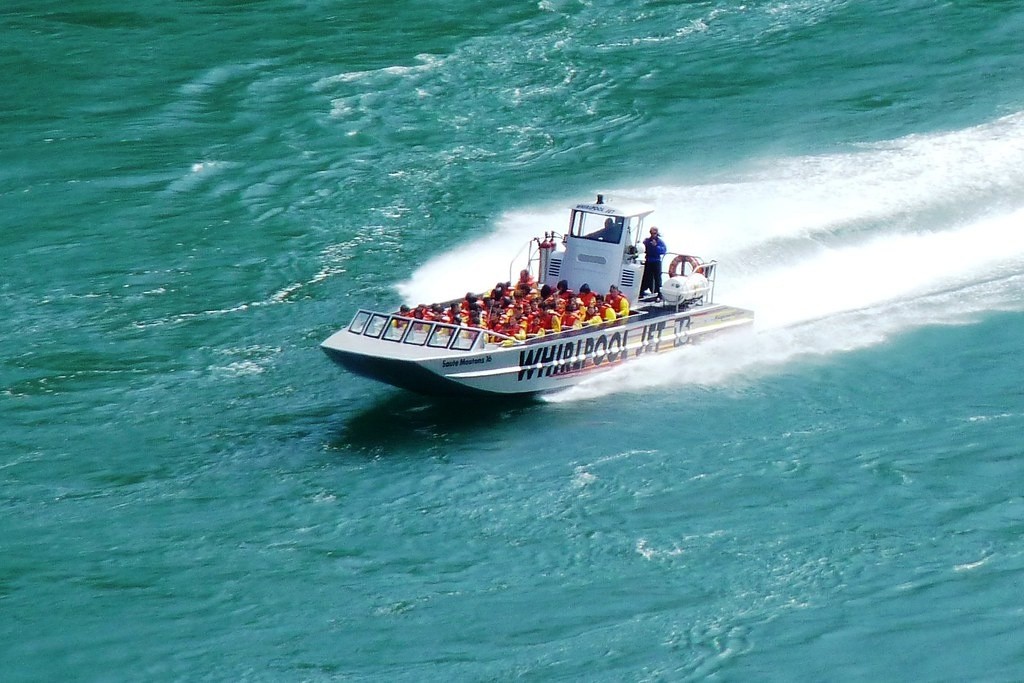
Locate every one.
[656,297,661,303]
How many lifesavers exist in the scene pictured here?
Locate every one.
[668,254,703,279]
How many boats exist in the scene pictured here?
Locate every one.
[320,193,753,405]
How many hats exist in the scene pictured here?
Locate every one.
[650,226,659,232]
[565,305,573,311]
[595,294,605,301]
[610,284,619,290]
[580,283,591,293]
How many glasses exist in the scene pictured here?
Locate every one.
[515,307,523,310]
[609,290,617,293]
[651,233,658,234]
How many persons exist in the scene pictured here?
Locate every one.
[639,226,666,303]
[392,270,630,348]
[591,218,619,243]
